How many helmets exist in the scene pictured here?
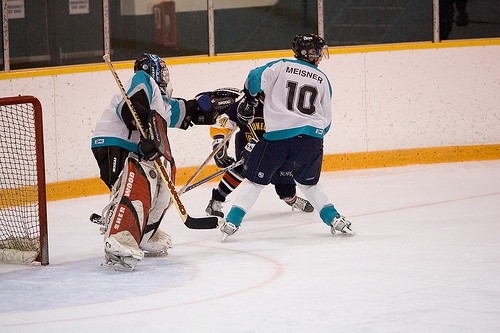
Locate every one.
[134,54,170,94]
[292,33,330,65]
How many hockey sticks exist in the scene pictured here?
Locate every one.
[103,53,225,230]
[171,125,238,203]
[89,158,244,226]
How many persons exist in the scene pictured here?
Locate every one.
[219,32,353,243]
[90,51,240,272]
[204,77,314,229]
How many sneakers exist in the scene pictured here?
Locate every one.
[205,200,224,221]
[286,196,314,212]
[221,221,239,243]
[100,250,140,272]
[330,216,354,236]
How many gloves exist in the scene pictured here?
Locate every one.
[212,139,236,168]
[237,96,259,125]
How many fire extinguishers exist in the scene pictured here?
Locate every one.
[164,7,171,38]
